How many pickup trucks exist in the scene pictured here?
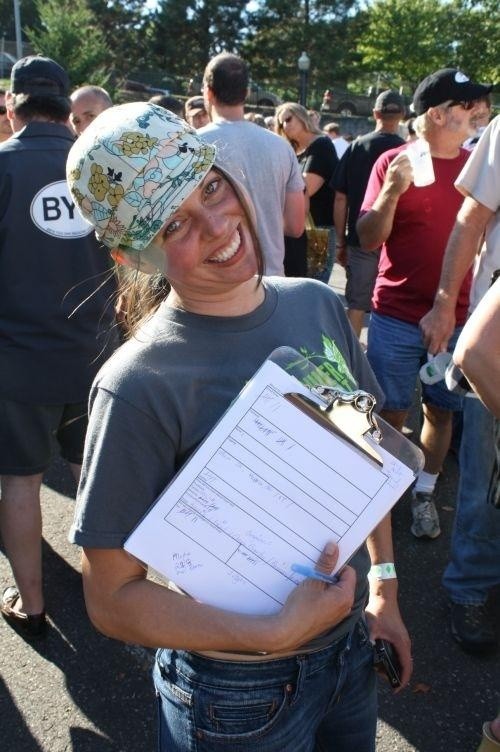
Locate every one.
[322,87,409,118]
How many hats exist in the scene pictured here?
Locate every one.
[414,68,493,116]
[185,96,206,117]
[65,102,217,252]
[10,54,70,96]
[375,90,404,113]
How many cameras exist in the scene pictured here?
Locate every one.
[420,352,451,385]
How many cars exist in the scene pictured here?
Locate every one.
[240,76,279,107]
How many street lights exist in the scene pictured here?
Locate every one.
[294,50,315,107]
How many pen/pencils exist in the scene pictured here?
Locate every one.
[292,564,337,586]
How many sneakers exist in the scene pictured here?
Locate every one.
[1,585,46,641]
[440,473,459,495]
[450,600,497,649]
[410,491,442,541]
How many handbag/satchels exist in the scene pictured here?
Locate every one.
[305,212,331,274]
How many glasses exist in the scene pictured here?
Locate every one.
[448,97,475,110]
[0,105,7,115]
[279,114,296,129]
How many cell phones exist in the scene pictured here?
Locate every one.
[374,639,400,688]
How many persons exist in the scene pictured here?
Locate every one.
[63,99,414,750]
[0,52,499,660]
